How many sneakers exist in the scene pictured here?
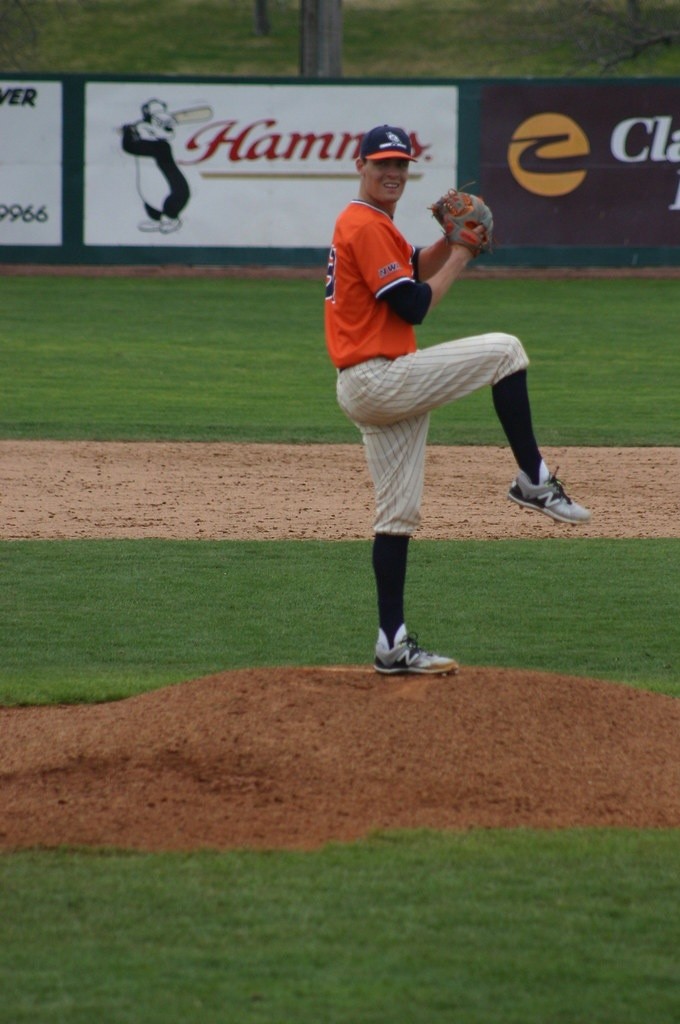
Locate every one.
[372,639,459,677]
[506,472,590,527]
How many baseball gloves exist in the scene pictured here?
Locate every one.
[429,188,495,257]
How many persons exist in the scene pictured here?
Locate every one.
[323,124,592,676]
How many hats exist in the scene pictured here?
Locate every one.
[360,125,418,165]
